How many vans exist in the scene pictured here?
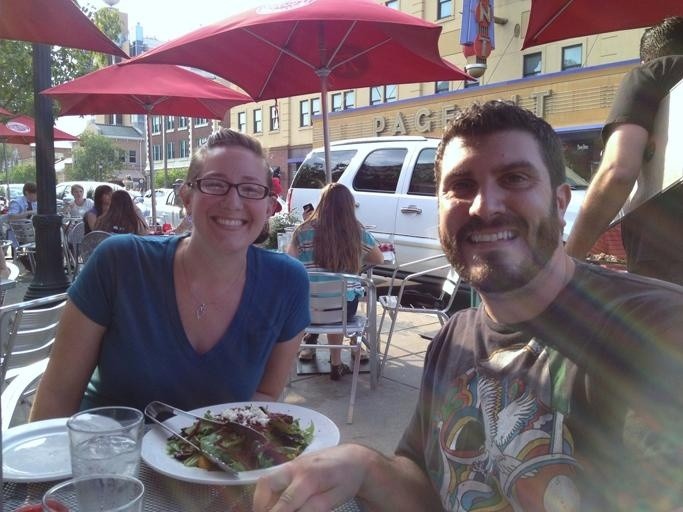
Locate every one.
[285,135,615,298]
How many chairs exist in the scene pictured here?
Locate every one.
[300,271,377,424]
[0,205,112,283]
[1,290,71,429]
[376,252,462,380]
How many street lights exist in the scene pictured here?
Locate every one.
[99,162,104,181]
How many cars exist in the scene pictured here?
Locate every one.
[0,180,286,230]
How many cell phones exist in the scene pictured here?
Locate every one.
[303,203,314,211]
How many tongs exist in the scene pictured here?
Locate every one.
[142,400,268,478]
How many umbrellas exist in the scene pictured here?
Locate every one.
[120,0,477,184]
[39,57,248,222]
[0,106,82,144]
[0,2,132,60]
[520,1,683,50]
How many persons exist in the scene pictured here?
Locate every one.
[284,182,385,382]
[1,180,37,273]
[562,14,682,287]
[27,127,313,423]
[82,184,112,235]
[93,191,148,235]
[250,95,681,511]
[296,325,370,362]
[62,184,93,274]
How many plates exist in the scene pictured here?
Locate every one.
[1,414,122,485]
[139,401,342,487]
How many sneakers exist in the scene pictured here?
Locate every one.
[350,340,369,362]
[298,332,316,362]
[328,359,351,379]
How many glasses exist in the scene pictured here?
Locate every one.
[187,176,273,200]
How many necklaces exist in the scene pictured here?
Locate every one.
[178,244,245,322]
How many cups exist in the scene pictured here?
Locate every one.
[276,232,291,252]
[65,404,144,512]
[42,477,145,512]
[284,226,297,241]
